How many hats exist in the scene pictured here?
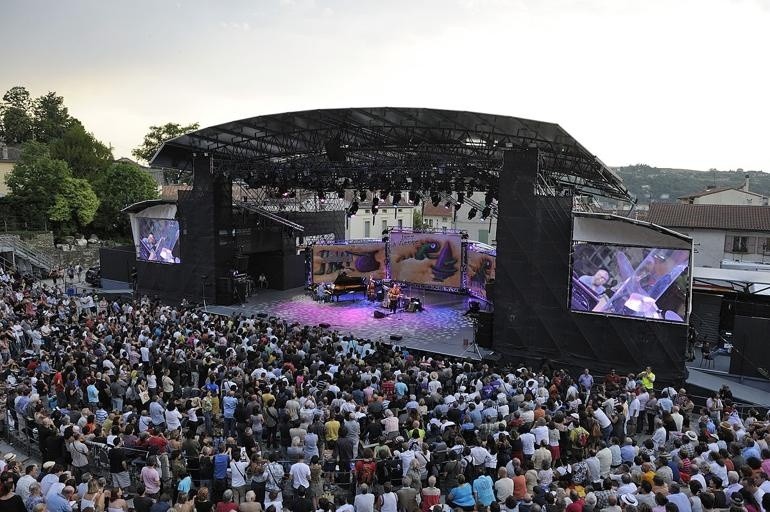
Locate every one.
[728,491,744,505]
[621,493,638,507]
[3,452,16,462]
[719,420,734,430]
[710,433,719,441]
[685,430,698,440]
[429,371,439,381]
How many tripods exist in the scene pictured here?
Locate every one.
[198,284,210,311]
[461,321,483,360]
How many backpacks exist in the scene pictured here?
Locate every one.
[573,427,587,447]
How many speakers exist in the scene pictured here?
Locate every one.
[476,310,492,348]
[216,276,230,305]
[485,285,495,300]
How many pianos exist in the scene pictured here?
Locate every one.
[333,274,367,302]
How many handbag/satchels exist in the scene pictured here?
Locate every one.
[138,387,150,405]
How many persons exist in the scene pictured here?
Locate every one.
[578,269,609,295]
[143,231,156,250]
[131,266,137,289]
[49,263,83,284]
[1,273,769,512]
